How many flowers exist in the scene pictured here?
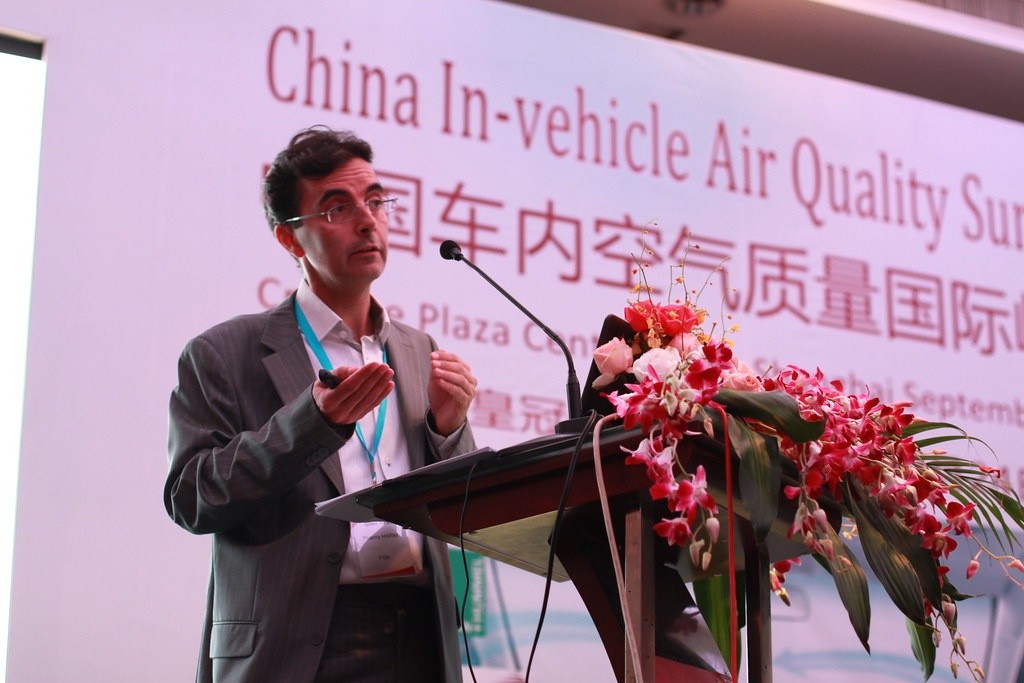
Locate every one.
[591,215,1024,683]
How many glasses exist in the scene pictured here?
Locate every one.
[282,195,398,225]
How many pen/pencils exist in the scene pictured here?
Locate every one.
[318,367,339,387]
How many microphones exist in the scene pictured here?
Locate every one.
[439,240,611,431]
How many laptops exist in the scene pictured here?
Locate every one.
[582,314,639,424]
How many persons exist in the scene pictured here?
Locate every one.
[162,124,479,683]
